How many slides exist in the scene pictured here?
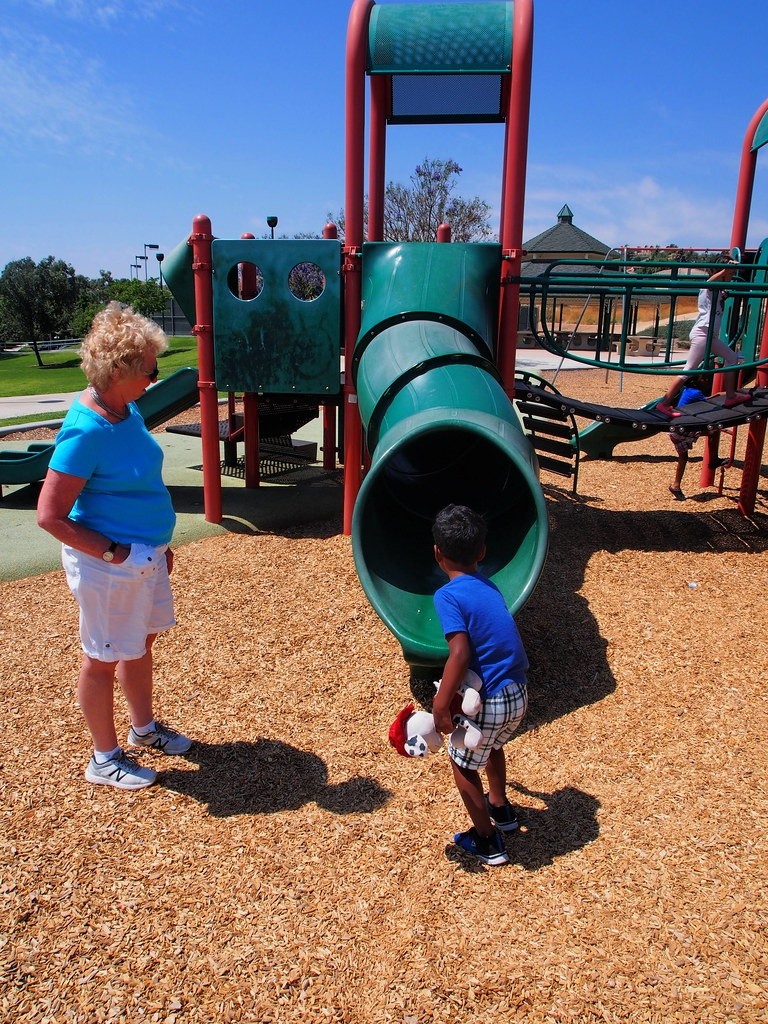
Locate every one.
[570,394,668,460]
[349,242,556,666]
[0,368,200,487]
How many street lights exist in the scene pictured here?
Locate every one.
[143,243,160,320]
[155,253,165,335]
[267,215,278,239]
[135,254,148,314]
[130,263,141,308]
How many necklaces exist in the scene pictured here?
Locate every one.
[87,386,128,419]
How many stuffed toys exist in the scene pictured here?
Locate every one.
[388,668,483,758]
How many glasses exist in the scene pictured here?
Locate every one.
[141,367,160,383]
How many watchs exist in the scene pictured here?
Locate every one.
[102,542,118,563]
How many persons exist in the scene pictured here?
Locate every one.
[36,301,193,791]
[433,504,530,866]
[656,256,752,418]
[669,376,729,502]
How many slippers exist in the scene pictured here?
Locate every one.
[708,457,730,469]
[668,485,686,502]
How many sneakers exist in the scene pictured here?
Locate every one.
[85,749,157,789]
[454,826,508,865]
[127,721,192,754]
[484,792,518,831]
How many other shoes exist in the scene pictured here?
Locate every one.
[724,393,750,406]
[656,402,682,417]
